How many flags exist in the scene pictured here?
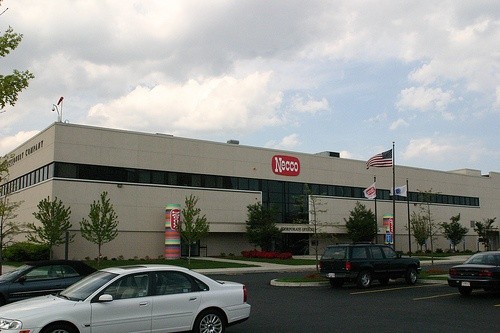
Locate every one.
[363,183,376,200]
[390,185,407,198]
[364,149,393,170]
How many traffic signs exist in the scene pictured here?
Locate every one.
[386,233,392,245]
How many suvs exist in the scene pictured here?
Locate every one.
[318,242,423,291]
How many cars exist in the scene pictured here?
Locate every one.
[0,264,252,333]
[0,259,98,305]
[448,250,500,301]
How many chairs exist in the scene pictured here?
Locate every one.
[120,277,138,298]
